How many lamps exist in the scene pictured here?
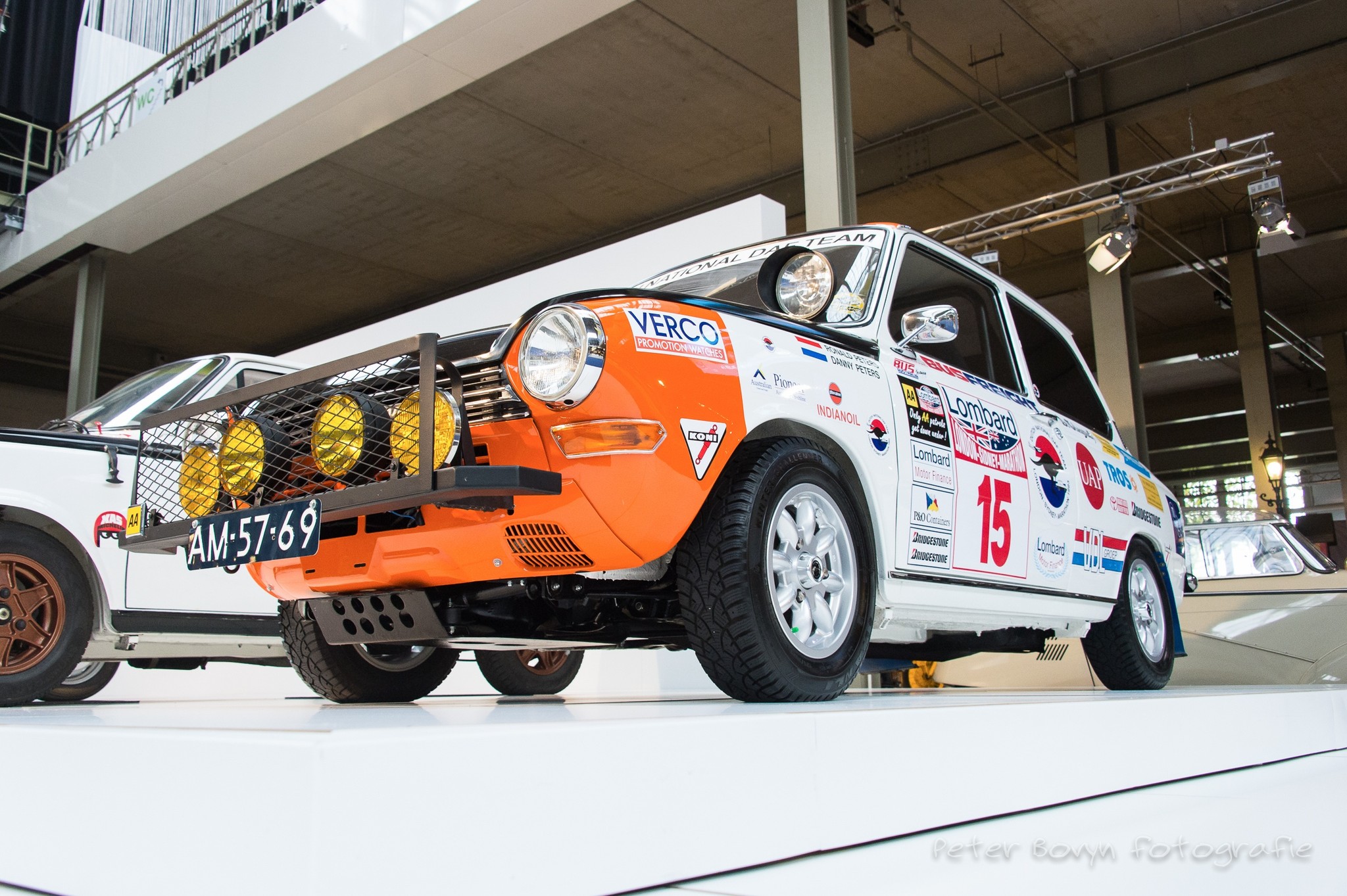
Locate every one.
[1082,203,1140,276]
[1247,171,1307,256]
[1259,431,1296,515]
[4,198,25,230]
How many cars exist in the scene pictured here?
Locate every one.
[930,506,1347,687]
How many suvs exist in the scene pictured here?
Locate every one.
[0,351,310,707]
[119,222,1199,703]
